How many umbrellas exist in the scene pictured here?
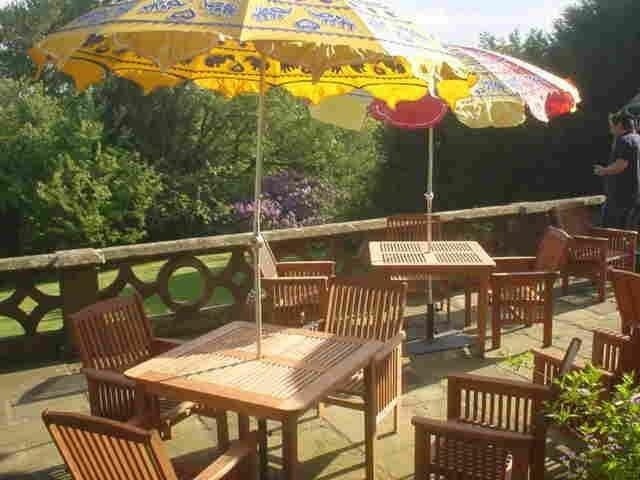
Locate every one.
[24,1,483,357]
[301,40,583,304]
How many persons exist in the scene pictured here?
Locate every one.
[592,111,640,234]
